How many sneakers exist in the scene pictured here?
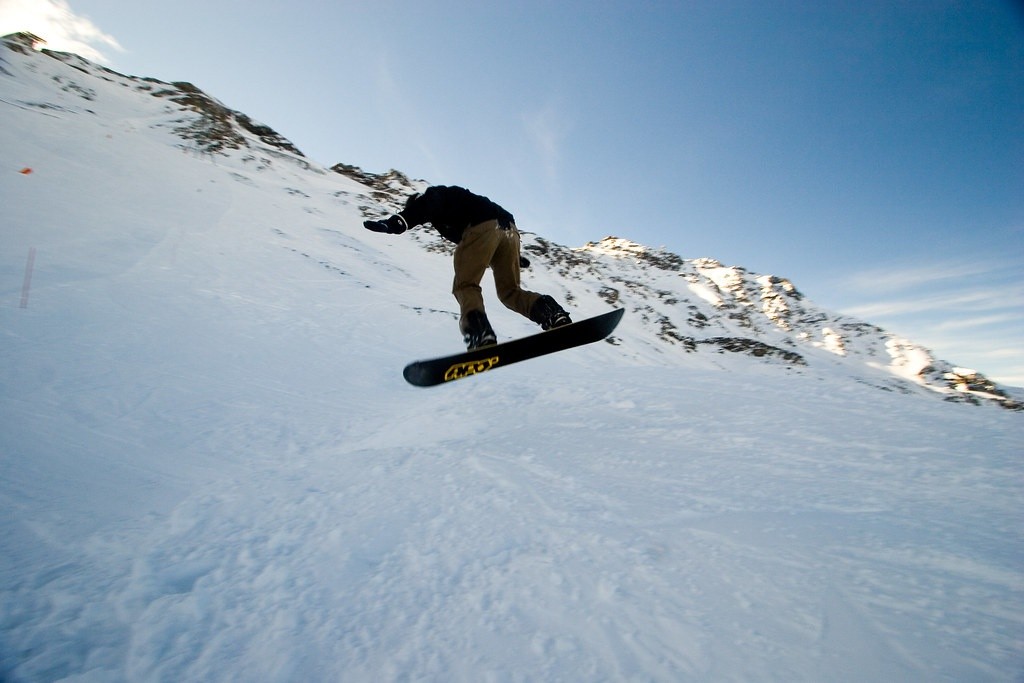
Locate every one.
[546,309,572,331]
[464,330,496,351]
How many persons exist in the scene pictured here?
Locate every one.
[364,184,572,350]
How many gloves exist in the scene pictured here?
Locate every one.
[364,220,392,234]
[520,256,530,268]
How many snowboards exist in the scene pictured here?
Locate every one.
[401,305,625,387]
